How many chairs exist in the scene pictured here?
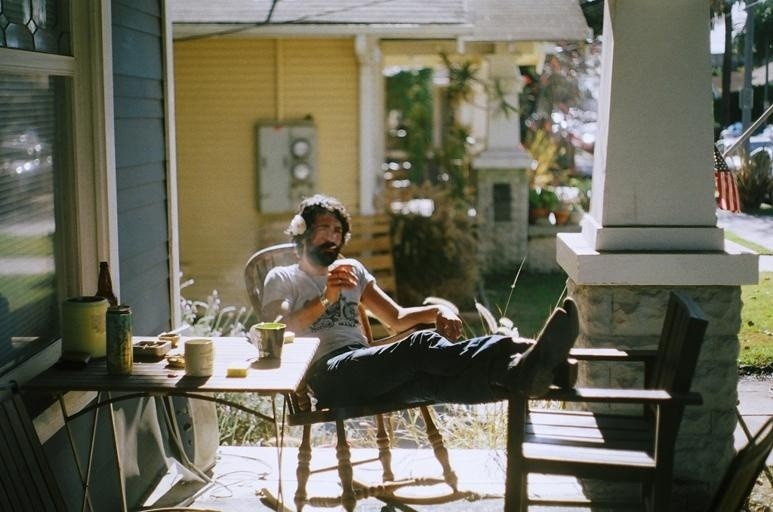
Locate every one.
[244,243,479,512]
[505,292,709,512]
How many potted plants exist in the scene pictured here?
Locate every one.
[530,187,550,222]
[551,193,573,225]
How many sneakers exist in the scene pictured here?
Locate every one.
[512,297,582,398]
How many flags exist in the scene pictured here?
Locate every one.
[713,145,741,214]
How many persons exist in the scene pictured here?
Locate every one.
[259,193,580,398]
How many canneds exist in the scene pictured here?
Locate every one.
[106,305,133,377]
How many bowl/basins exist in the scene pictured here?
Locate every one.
[157,333,181,349]
[281,331,295,344]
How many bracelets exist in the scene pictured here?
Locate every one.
[317,296,332,308]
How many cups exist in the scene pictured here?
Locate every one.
[184,339,213,377]
[255,323,287,362]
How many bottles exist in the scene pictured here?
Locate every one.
[94,261,117,315]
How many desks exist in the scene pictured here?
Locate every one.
[17,336,322,511]
